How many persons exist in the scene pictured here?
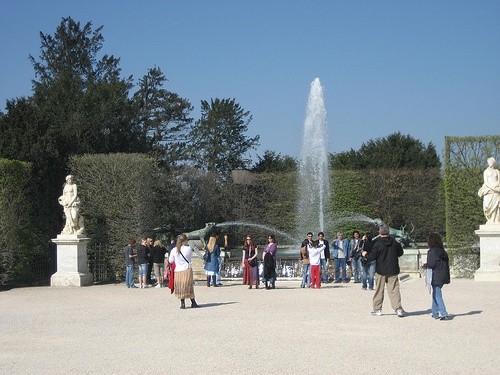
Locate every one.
[202,232,230,287]
[477,156,500,224]
[262,234,277,289]
[123,234,169,290]
[240,234,261,289]
[165,234,200,308]
[421,231,451,319]
[58,174,82,234]
[300,225,407,318]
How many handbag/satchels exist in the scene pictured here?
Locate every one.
[203,248,209,261]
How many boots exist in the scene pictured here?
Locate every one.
[206,277,210,287]
[192,299,197,307]
[180,299,185,309]
[212,275,218,287]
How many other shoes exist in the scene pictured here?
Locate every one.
[256,286,259,289]
[398,311,405,317]
[441,316,450,320]
[432,315,439,319]
[248,286,252,289]
[143,285,150,288]
[155,285,163,288]
[140,285,143,288]
[374,311,381,316]
[130,285,138,288]
